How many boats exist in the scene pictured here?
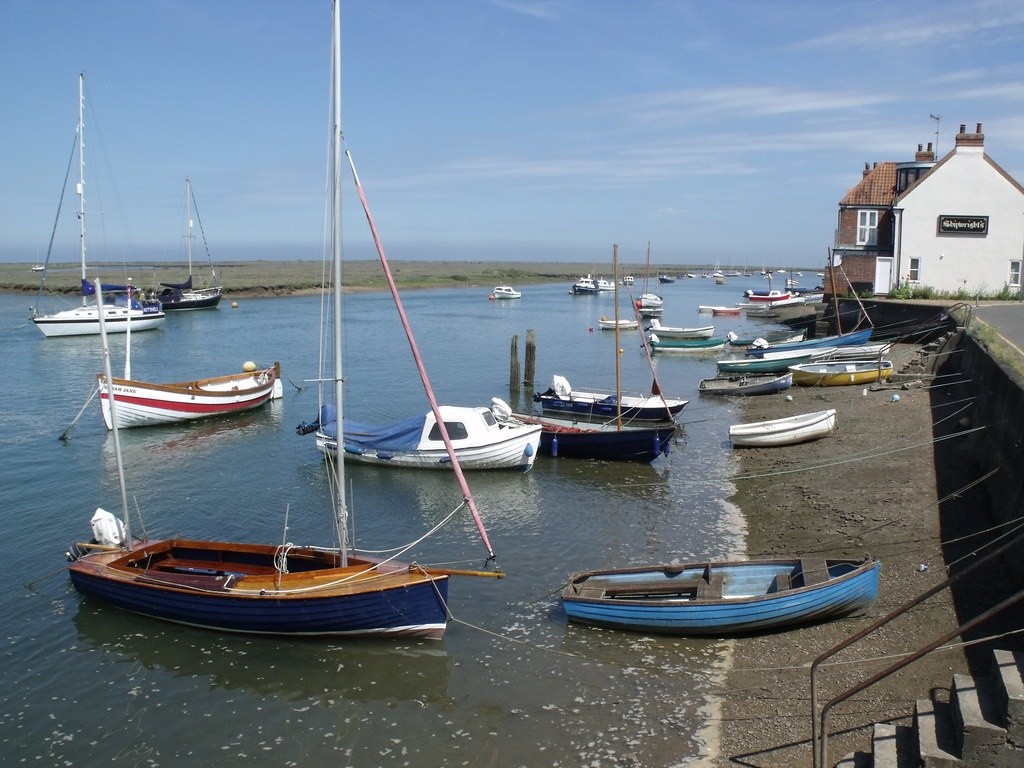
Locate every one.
[698,373,792,396]
[536,384,690,424]
[717,347,838,374]
[686,265,824,289]
[598,317,638,331]
[696,306,744,316]
[786,360,893,387]
[297,403,544,472]
[638,308,665,317]
[639,332,730,354]
[745,289,790,300]
[493,286,521,300]
[94,277,285,432]
[566,557,882,639]
[643,319,715,341]
[658,275,675,284]
[763,341,891,360]
[727,328,810,349]
[623,275,634,285]
[728,409,839,449]
[32,264,45,272]
[785,286,816,295]
[573,274,617,291]
[569,285,600,295]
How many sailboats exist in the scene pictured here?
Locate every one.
[148,176,224,311]
[491,244,682,466]
[634,240,663,308]
[747,247,875,355]
[59,0,508,641]
[28,74,168,339]
